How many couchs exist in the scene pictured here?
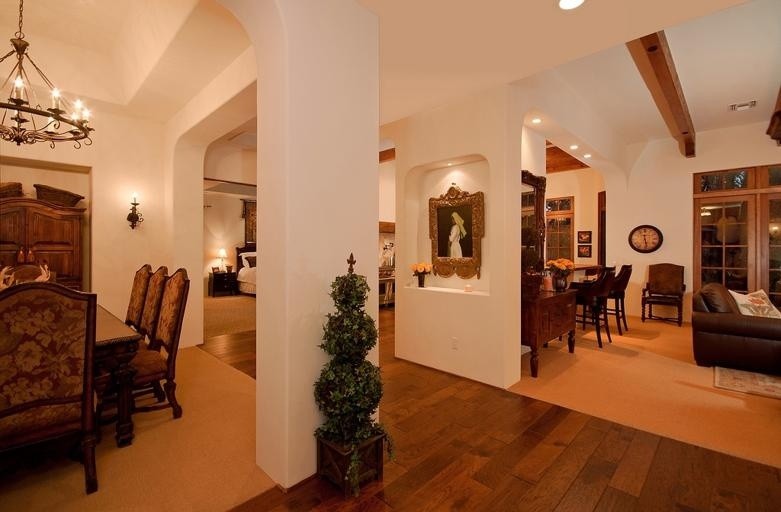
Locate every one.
[690,286,781,377]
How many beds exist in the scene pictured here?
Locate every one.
[236,247,256,298]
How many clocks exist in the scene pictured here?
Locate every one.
[629,225,663,255]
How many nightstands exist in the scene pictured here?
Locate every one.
[208,272,239,296]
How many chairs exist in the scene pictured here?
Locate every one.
[568,268,612,348]
[0,280,102,495]
[582,264,633,336]
[640,264,687,326]
[121,264,153,336]
[113,265,167,355]
[93,268,191,435]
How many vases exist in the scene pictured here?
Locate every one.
[552,274,567,293]
[417,273,425,287]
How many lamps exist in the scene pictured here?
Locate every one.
[125,191,143,229]
[1,0,94,150]
[217,249,228,273]
[559,0,586,11]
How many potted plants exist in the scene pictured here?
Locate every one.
[521,228,544,299]
[315,252,395,500]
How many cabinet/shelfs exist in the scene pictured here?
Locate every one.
[1,198,87,289]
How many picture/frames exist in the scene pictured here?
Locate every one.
[578,231,592,244]
[578,245,592,258]
[428,186,485,279]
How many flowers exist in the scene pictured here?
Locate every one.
[411,263,431,274]
[546,256,576,277]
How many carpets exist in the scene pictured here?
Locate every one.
[714,364,780,400]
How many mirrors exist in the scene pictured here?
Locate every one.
[522,167,546,274]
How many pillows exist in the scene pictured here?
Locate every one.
[701,284,742,315]
[729,288,781,318]
